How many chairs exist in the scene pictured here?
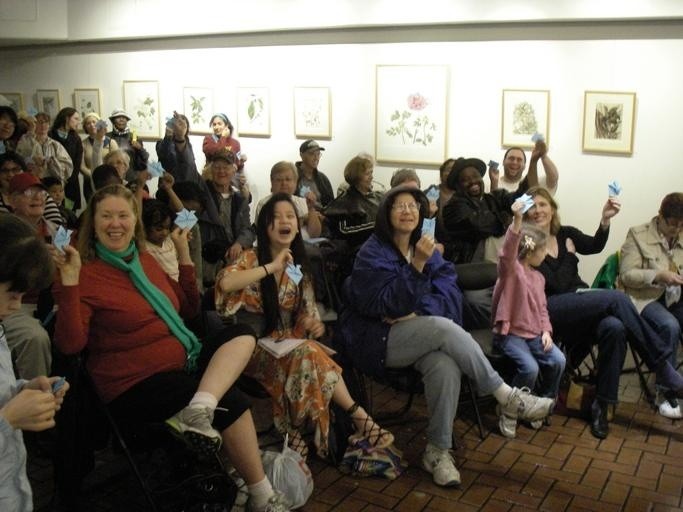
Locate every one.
[555,339,599,382]
[46,311,247,510]
[337,277,457,451]
[455,261,553,438]
[597,254,683,412]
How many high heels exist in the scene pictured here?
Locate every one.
[587,397,609,439]
[659,385,683,408]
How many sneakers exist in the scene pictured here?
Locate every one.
[495,386,557,423]
[495,403,519,439]
[653,388,682,419]
[243,489,291,512]
[530,419,543,429]
[421,442,463,487]
[163,404,223,456]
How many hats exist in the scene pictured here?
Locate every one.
[6,172,48,196]
[300,139,326,154]
[445,156,487,192]
[212,148,237,164]
[109,108,131,121]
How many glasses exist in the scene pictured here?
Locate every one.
[0,167,23,175]
[663,215,683,229]
[13,188,48,200]
[391,200,422,213]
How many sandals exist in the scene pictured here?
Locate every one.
[345,400,396,449]
[282,427,310,463]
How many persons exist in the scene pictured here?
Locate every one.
[0,105,680,512]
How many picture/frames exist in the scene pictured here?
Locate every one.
[74,89,101,132]
[122,79,162,141]
[293,87,332,140]
[582,91,636,157]
[182,87,216,136]
[502,89,550,151]
[236,86,271,138]
[36,89,61,122]
[0,93,23,114]
[375,65,449,169]
